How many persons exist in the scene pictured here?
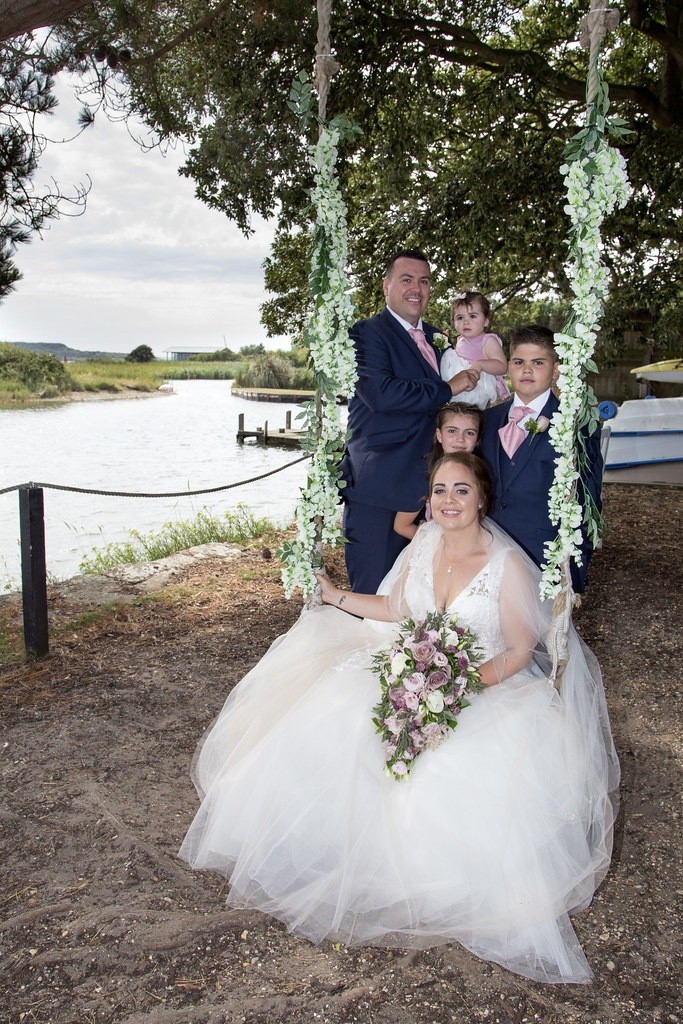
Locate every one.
[477,325,603,593]
[425,401,485,477]
[440,291,511,411]
[175,450,620,983]
[336,250,481,622]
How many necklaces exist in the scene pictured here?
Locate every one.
[441,549,475,575]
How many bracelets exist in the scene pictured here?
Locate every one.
[335,592,349,607]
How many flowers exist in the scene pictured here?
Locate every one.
[524,415,549,447]
[433,333,451,355]
[454,292,466,300]
[272,71,366,598]
[367,612,485,783]
[537,48,637,605]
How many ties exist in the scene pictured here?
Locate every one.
[409,329,439,375]
[498,407,533,460]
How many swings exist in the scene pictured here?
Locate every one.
[273,0,629,705]
